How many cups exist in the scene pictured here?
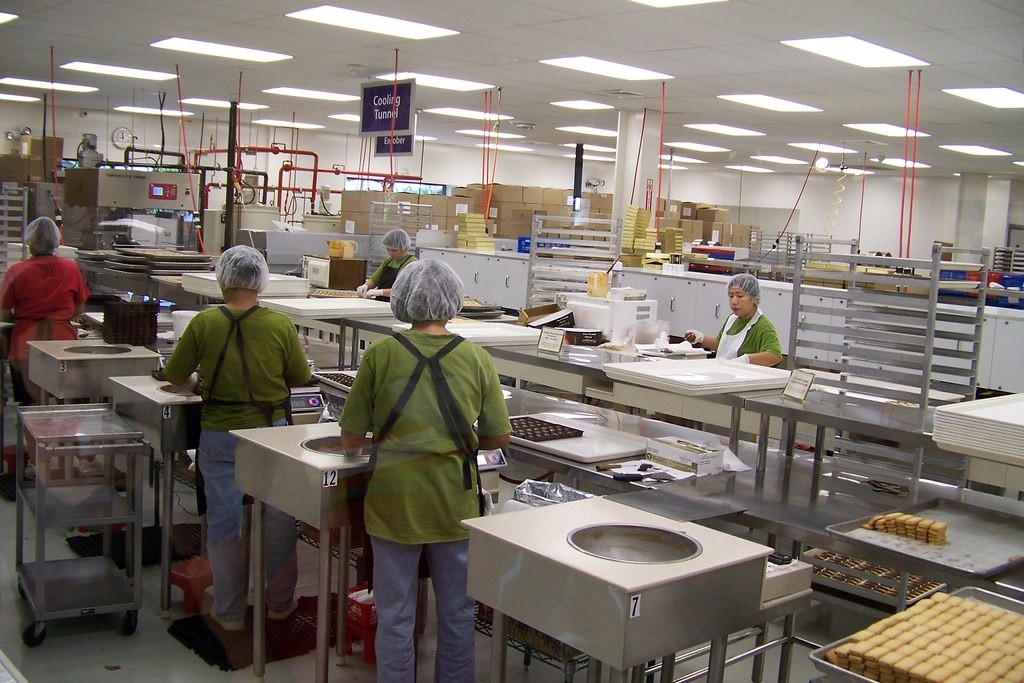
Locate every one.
[586,272,611,297]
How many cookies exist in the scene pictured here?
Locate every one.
[826,591,1024,683]
[863,513,947,545]
[810,550,940,600]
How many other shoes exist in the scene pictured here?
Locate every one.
[80,458,104,477]
[267,593,298,619]
[211,606,246,631]
[24,459,33,479]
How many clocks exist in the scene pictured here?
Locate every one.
[112,127,133,150]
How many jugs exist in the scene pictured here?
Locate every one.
[326,240,345,259]
[342,240,359,260]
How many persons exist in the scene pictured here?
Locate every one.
[0,217,101,480]
[164,246,311,631]
[356,230,417,303]
[338,261,512,683]
[683,276,781,365]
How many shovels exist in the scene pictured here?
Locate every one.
[613,471,677,481]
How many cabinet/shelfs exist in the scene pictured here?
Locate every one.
[16,403,147,647]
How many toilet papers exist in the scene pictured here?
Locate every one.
[611,301,637,344]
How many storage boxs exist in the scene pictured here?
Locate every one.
[341,183,760,253]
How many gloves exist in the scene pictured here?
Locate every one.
[729,354,749,364]
[683,330,704,344]
[366,289,383,298]
[357,284,368,297]
[189,372,204,395]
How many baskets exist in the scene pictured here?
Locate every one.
[348,584,377,630]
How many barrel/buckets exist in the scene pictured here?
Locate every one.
[173,310,202,341]
